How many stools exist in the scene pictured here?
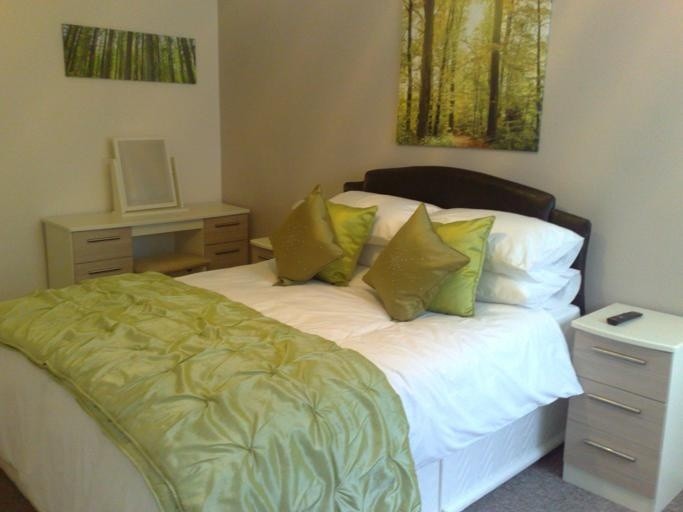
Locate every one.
[134,251,210,277]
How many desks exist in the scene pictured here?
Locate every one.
[41,201,250,288]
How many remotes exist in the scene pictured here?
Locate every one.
[606,310,643,325]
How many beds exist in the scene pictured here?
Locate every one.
[0,164,591,511]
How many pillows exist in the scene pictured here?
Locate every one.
[429,207,584,283]
[477,270,582,312]
[269,183,346,286]
[357,244,384,267]
[427,215,496,318]
[362,201,471,323]
[315,200,377,288]
[328,190,440,243]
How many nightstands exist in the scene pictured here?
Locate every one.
[561,301,683,512]
